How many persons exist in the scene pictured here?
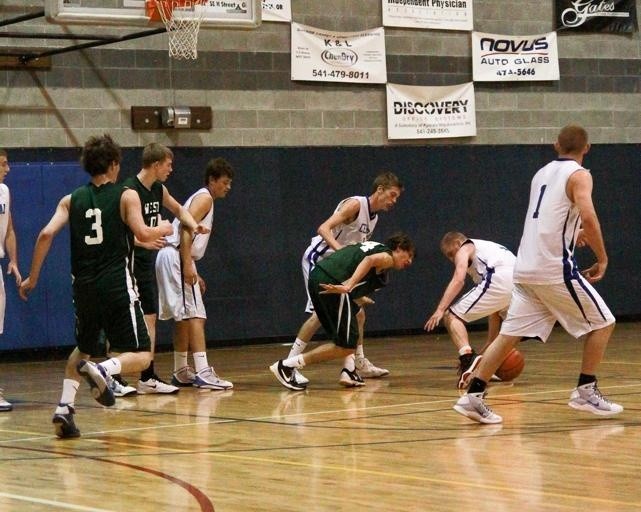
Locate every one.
[0,148,22,412]
[422,230,518,390]
[287,170,406,385]
[106,142,212,397]
[271,233,418,390]
[155,157,234,391]
[20,133,175,438]
[451,124,625,423]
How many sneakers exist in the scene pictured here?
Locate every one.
[136,374,180,395]
[355,358,390,379]
[193,366,233,390]
[452,390,502,424]
[111,372,138,397]
[568,380,623,416]
[0,388,12,412]
[268,359,306,391]
[455,349,483,389]
[77,359,115,407]
[339,367,365,387]
[295,369,309,384]
[172,366,195,387]
[52,405,81,439]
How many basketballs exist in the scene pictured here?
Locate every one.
[497,348,524,381]
